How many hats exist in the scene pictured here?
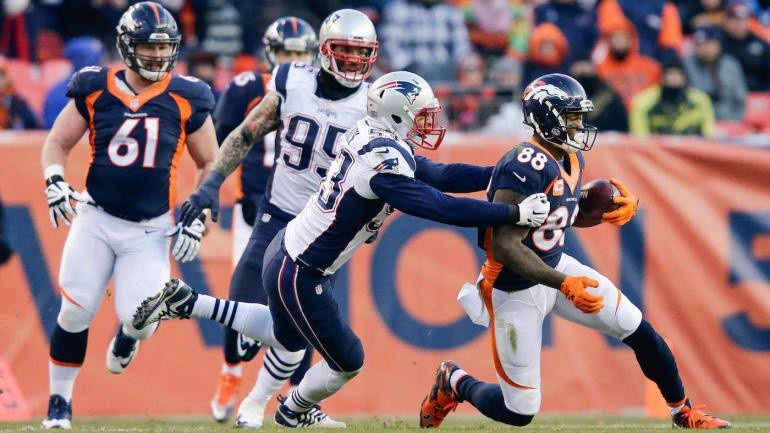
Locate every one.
[65,36,106,71]
[726,2,753,20]
[658,46,687,71]
[692,25,724,44]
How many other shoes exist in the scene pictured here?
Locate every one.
[235,396,265,429]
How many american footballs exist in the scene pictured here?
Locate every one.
[579,178,621,221]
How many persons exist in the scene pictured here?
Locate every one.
[0,0,770,142]
[42,1,219,427]
[132,8,550,430]
[419,74,732,431]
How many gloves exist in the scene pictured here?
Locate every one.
[164,209,209,264]
[177,169,227,228]
[516,192,551,228]
[44,174,86,229]
[602,178,638,227]
[560,275,605,314]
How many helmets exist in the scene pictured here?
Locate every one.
[320,9,377,88]
[262,17,318,73]
[367,71,439,149]
[116,2,178,80]
[521,73,595,154]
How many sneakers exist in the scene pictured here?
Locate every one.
[42,394,73,430]
[420,360,464,428]
[105,324,140,375]
[273,393,346,428]
[672,398,731,430]
[211,372,241,423]
[131,277,199,331]
